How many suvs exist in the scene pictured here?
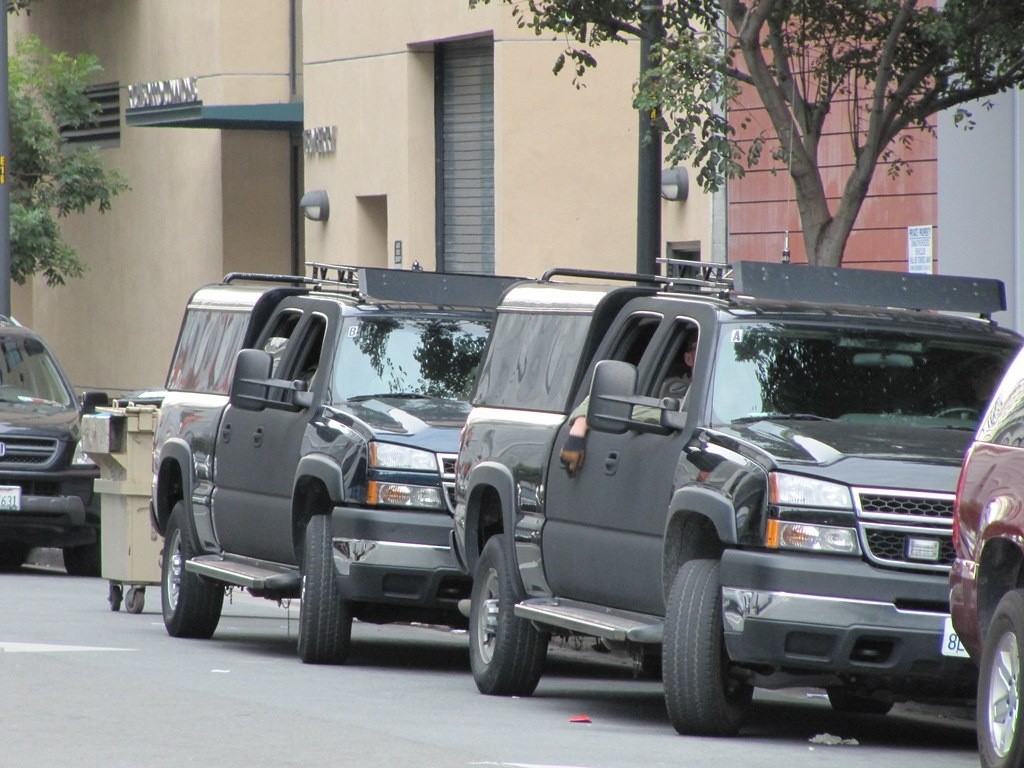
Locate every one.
[944,338,1023,768]
[144,261,541,668]
[1,313,110,581]
[448,261,1023,739]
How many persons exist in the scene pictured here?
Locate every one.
[558,328,700,478]
